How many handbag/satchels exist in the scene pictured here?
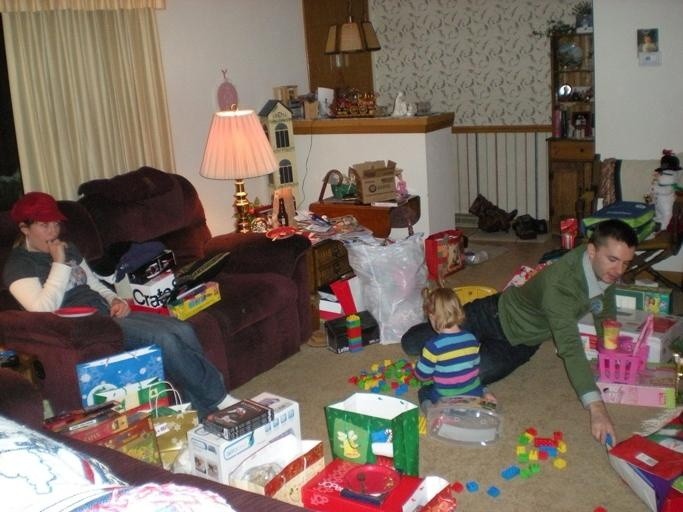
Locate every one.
[227,434,326,508]
[148,381,199,471]
[324,392,418,476]
[425,230,465,279]
[76,346,164,408]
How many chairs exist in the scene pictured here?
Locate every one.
[575,157,683,293]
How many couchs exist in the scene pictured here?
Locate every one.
[0,173,313,417]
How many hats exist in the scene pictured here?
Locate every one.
[11,192,67,223]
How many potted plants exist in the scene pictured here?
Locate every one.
[571,1,592,34]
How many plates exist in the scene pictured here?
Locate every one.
[265,226,296,240]
[342,464,401,495]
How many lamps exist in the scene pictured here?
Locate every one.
[324,15,382,74]
[198,104,281,235]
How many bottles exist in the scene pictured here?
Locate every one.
[277,198,288,227]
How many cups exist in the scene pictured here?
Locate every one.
[52,305,97,318]
[601,318,622,350]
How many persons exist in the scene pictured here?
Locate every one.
[637,30,657,53]
[4,191,243,424]
[412,287,495,413]
[401,217,638,449]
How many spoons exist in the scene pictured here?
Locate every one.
[357,473,366,496]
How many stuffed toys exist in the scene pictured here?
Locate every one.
[645,148,682,230]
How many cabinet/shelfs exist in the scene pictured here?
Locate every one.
[544,32,595,231]
[308,238,352,332]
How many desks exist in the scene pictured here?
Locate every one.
[308,193,420,245]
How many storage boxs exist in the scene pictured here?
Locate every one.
[553,308,682,363]
[185,391,301,487]
[348,160,397,205]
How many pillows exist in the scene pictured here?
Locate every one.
[1,415,235,511]
